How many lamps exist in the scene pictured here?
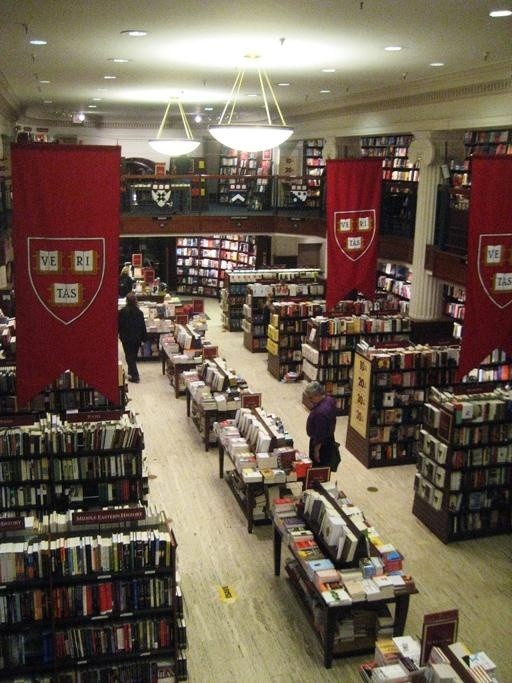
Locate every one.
[148,95,201,157]
[207,54,295,153]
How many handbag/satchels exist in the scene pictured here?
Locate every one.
[315,412,342,472]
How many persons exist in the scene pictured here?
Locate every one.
[119,262,133,297]
[118,292,145,383]
[305,380,341,472]
[171,155,193,215]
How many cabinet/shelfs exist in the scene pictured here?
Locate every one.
[451,129,512,204]
[179,356,264,452]
[174,236,262,299]
[345,338,462,469]
[357,642,500,683]
[302,139,326,207]
[0,360,128,427]
[137,300,207,358]
[0,511,189,683]
[158,323,227,399]
[375,260,413,314]
[218,144,273,206]
[131,281,167,303]
[300,312,410,416]
[272,480,419,670]
[213,406,313,533]
[241,280,326,353]
[266,297,401,381]
[411,378,512,545]
[219,267,322,332]
[360,134,420,194]
[441,283,465,338]
[0,410,151,543]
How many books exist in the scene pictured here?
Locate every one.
[305,136,324,206]
[212,409,312,484]
[284,559,395,642]
[443,284,465,340]
[1,480,141,508]
[136,301,208,357]
[2,616,175,670]
[0,505,163,536]
[441,130,512,210]
[242,283,325,349]
[0,454,142,483]
[119,361,129,394]
[226,470,304,515]
[1,576,174,625]
[370,636,497,683]
[414,386,512,534]
[0,412,140,456]
[0,659,175,683]
[133,279,171,301]
[193,161,206,199]
[461,349,510,385]
[164,324,214,393]
[141,424,150,507]
[271,482,417,607]
[176,570,188,681]
[177,236,255,297]
[266,300,371,383]
[0,529,171,585]
[219,268,319,329]
[182,356,255,446]
[358,340,461,462]
[31,370,110,413]
[375,263,411,314]
[302,313,412,411]
[0,367,17,414]
[219,149,271,210]
[361,136,418,195]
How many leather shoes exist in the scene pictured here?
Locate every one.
[129,376,140,384]
